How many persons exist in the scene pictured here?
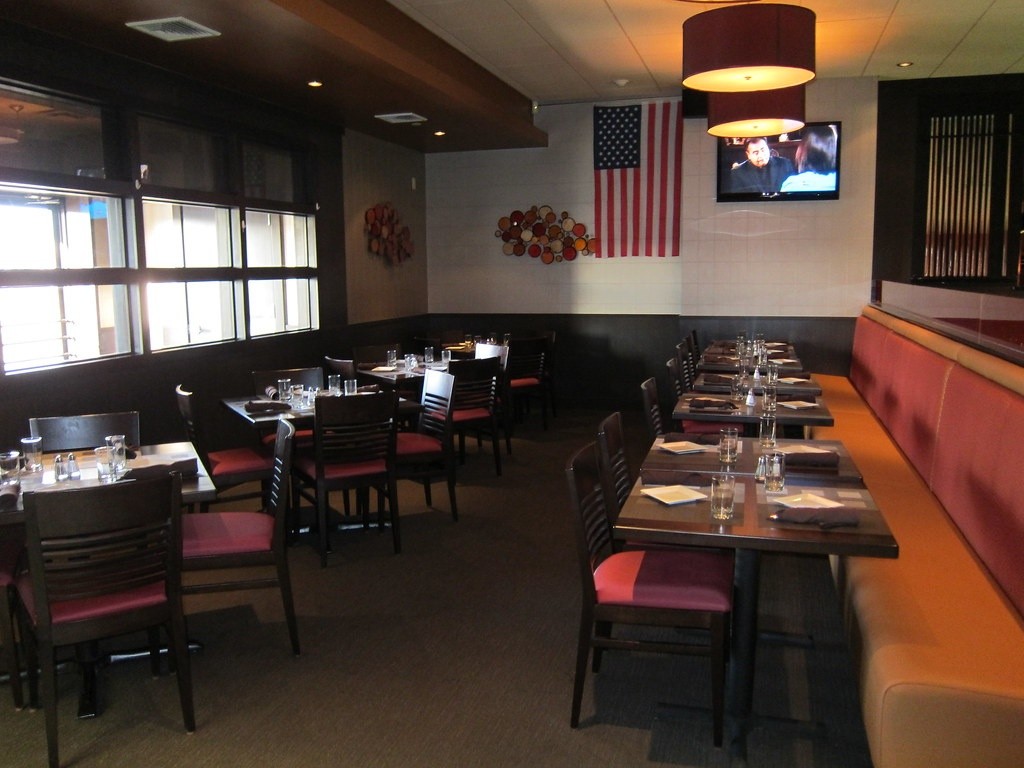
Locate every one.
[780,125,837,193]
[728,137,796,193]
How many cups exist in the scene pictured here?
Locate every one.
[385,347,454,372]
[0,433,126,487]
[463,332,510,350]
[760,416,776,448]
[711,475,735,520]
[277,374,358,410]
[766,451,786,492]
[720,426,737,463]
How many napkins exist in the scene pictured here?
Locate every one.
[703,356,734,363]
[775,508,861,528]
[0,456,29,472]
[767,344,788,352]
[115,458,199,481]
[356,363,387,371]
[769,393,816,403]
[0,479,20,508]
[638,470,720,488]
[763,353,791,360]
[110,434,137,460]
[265,386,280,400]
[772,451,840,468]
[244,400,292,413]
[703,374,733,385]
[687,399,738,411]
[663,432,725,445]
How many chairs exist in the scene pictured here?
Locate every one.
[566,327,736,747]
[0,330,558,768]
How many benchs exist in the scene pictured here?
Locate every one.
[809,305,1023,768]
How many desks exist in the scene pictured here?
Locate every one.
[695,339,802,374]
[220,389,425,555]
[693,373,822,403]
[0,441,216,720]
[613,475,898,768]
[355,357,451,384]
[639,433,863,483]
[672,391,835,440]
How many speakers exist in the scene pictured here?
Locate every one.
[681,83,708,119]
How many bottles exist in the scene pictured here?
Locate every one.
[731,330,779,411]
[755,456,765,482]
[52,453,63,481]
[67,452,80,479]
[745,388,757,406]
[752,364,760,379]
[752,347,759,357]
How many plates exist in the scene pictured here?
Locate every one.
[773,493,845,508]
[776,377,809,384]
[640,484,708,505]
[764,341,787,346]
[656,441,708,454]
[777,400,819,410]
[773,445,831,454]
[768,358,797,364]
[770,349,783,352]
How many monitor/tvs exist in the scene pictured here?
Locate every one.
[717,121,842,202]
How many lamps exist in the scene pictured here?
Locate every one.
[708,81,805,138]
[682,2,816,92]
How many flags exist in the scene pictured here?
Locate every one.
[592,100,684,257]
